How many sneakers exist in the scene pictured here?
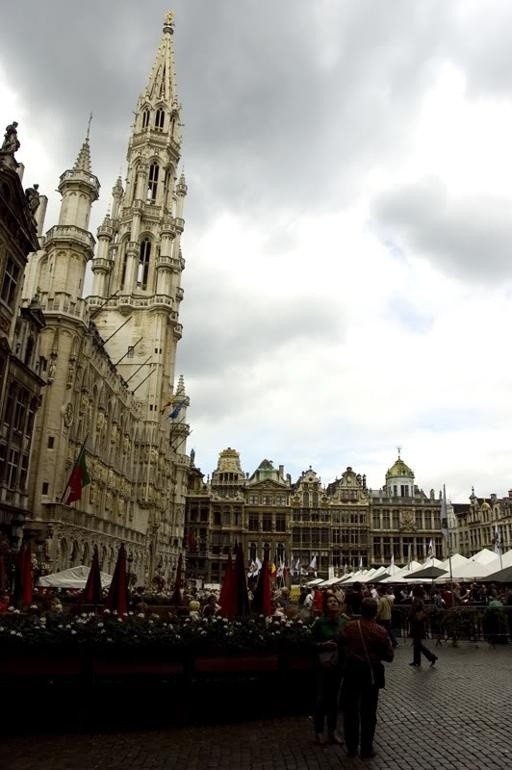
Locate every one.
[361,746,377,758]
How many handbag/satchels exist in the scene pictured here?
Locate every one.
[320,648,337,666]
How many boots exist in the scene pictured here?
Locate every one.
[316,730,344,746]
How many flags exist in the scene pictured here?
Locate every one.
[424,541,434,561]
[492,522,502,552]
[65,444,93,506]
[245,552,318,588]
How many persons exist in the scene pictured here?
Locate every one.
[305,593,344,746]
[337,597,396,759]
[0,581,512,668]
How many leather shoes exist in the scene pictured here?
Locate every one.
[410,662,420,666]
[430,656,438,666]
